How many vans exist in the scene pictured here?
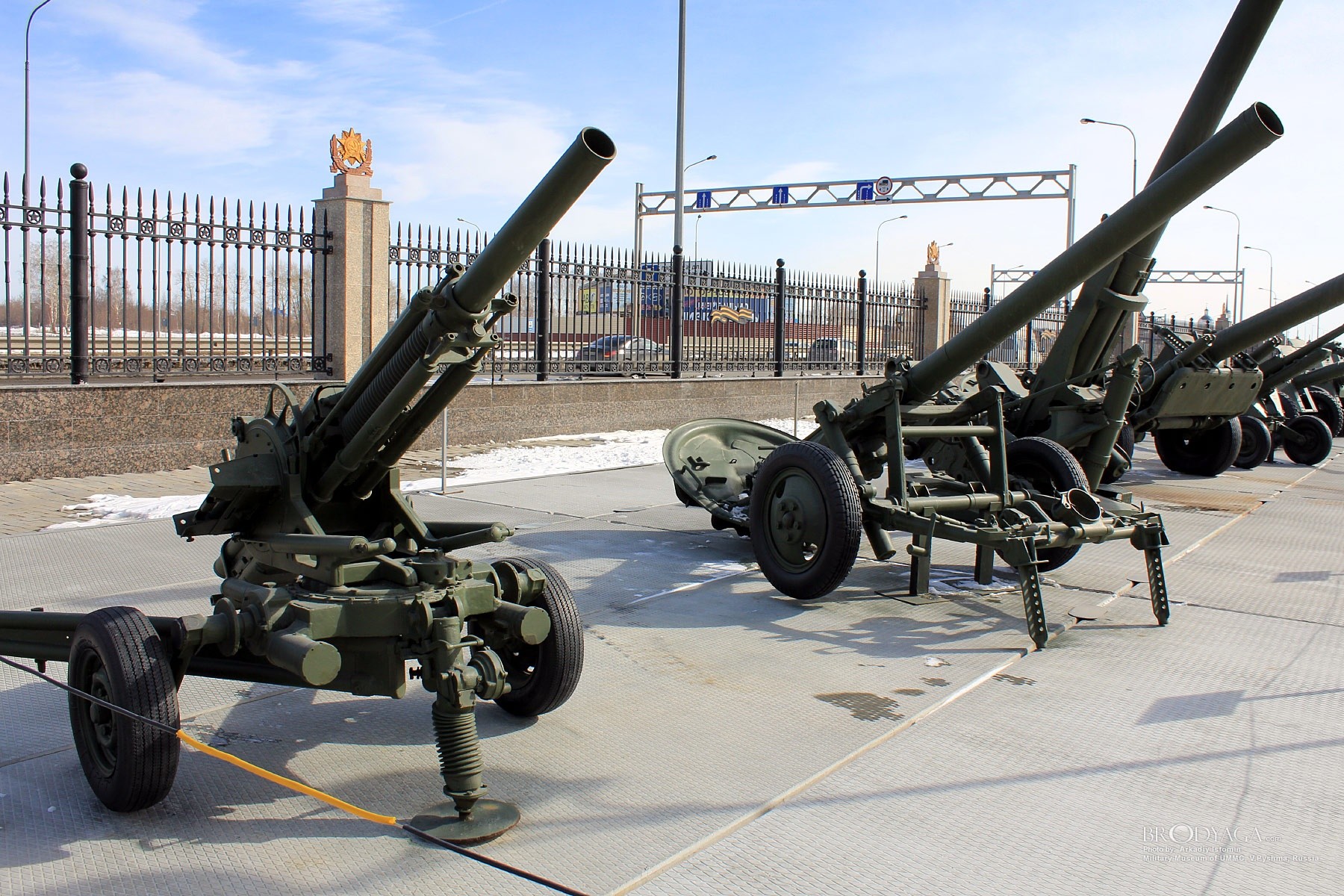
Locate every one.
[809,337,866,369]
[988,325,1041,368]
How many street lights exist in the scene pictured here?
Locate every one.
[1244,247,1298,346]
[457,219,481,255]
[1203,206,1240,326]
[1080,118,1136,197]
[694,215,701,275]
[992,265,1024,305]
[1306,281,1318,339]
[876,215,908,295]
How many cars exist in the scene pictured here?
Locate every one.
[575,335,669,376]
[767,342,811,359]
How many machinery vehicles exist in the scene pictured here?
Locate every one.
[1105,273,1344,478]
[0,126,617,848]
[662,101,1285,650]
[885,0,1284,495]
